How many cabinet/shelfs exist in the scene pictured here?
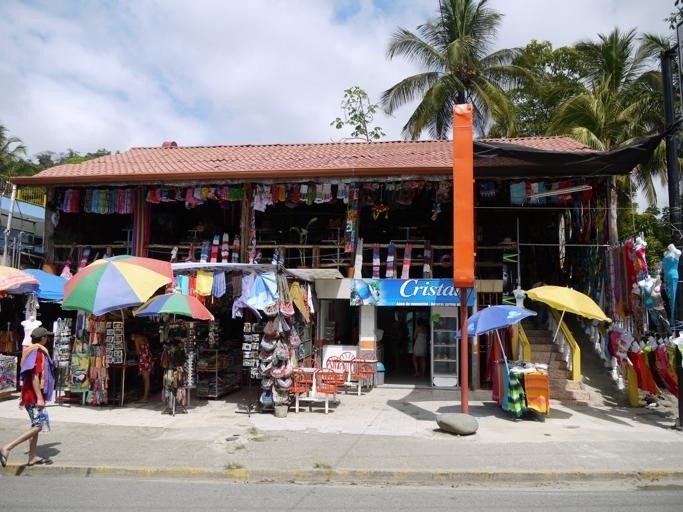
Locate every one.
[430,306,459,389]
[196,347,241,399]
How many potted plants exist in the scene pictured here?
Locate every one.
[288,216,318,268]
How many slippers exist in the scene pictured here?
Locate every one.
[0,447,8,467]
[27,456,53,466]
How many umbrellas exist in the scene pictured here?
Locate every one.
[524,284,611,342]
[59,254,172,320]
[453,303,538,369]
[131,289,216,323]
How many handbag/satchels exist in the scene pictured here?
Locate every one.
[255,298,304,408]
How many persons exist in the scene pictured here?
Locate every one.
[0,327,54,467]
[126,330,153,403]
[19,315,43,347]
[411,318,427,377]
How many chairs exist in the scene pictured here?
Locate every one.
[289,352,378,414]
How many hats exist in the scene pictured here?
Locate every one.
[30,327,54,339]
